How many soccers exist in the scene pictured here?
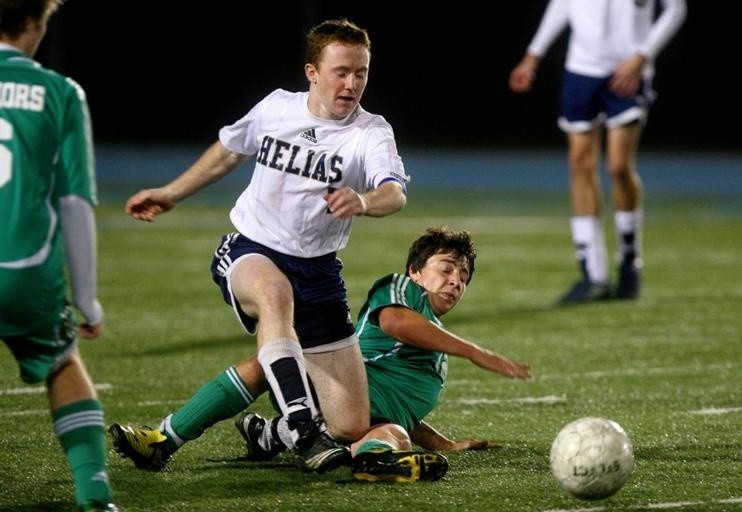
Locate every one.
[550,418,634,500]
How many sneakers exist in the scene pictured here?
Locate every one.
[548,282,611,306]
[348,448,450,485]
[232,409,282,460]
[291,430,349,475]
[109,421,172,473]
[612,255,645,300]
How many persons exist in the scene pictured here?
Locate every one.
[509,0,688,305]
[126,20,410,477]
[105,226,532,485]
[0,0,121,512]
